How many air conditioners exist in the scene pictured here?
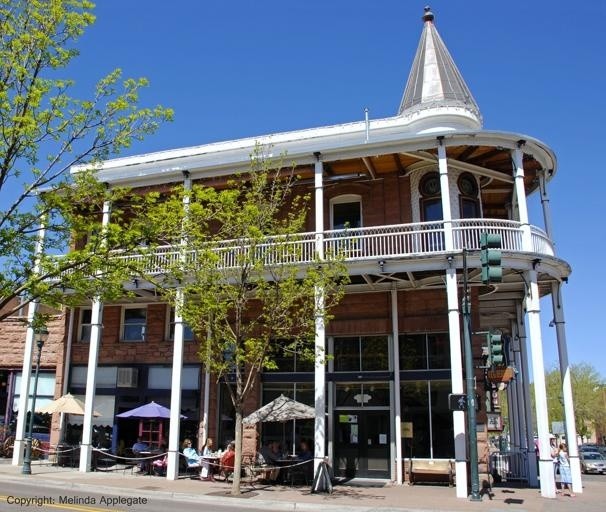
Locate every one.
[115,368,138,387]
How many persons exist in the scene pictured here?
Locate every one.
[182,438,211,481]
[558,442,577,497]
[220,442,234,472]
[132,435,149,473]
[550,432,563,494]
[299,441,312,460]
[265,440,283,483]
[202,437,213,455]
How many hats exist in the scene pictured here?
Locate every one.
[549,434,557,439]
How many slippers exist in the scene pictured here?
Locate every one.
[555,490,576,498]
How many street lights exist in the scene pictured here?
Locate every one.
[22,324,49,473]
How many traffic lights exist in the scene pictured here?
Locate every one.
[478,231,503,283]
[448,393,467,411]
[488,332,504,364]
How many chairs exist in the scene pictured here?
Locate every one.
[54,441,314,487]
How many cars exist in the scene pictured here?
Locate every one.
[25,425,49,441]
[578,443,606,474]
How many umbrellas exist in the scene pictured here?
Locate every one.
[39,392,104,440]
[243,390,330,444]
[114,398,189,449]
